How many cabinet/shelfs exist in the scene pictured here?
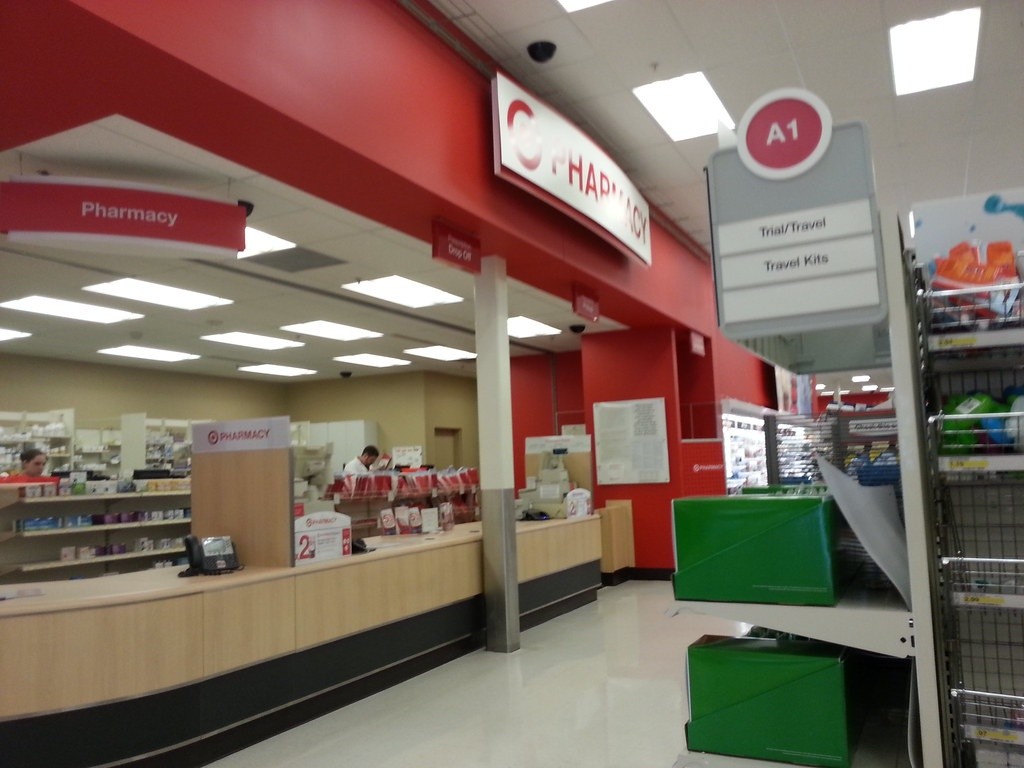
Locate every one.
[887,209,1024,768]
[311,419,378,484]
[316,481,480,553]
[0,489,191,581]
[0,407,299,484]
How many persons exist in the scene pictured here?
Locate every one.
[341,444,379,476]
[16,449,47,475]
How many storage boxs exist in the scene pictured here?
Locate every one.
[683,634,858,768]
[670,486,848,608]
